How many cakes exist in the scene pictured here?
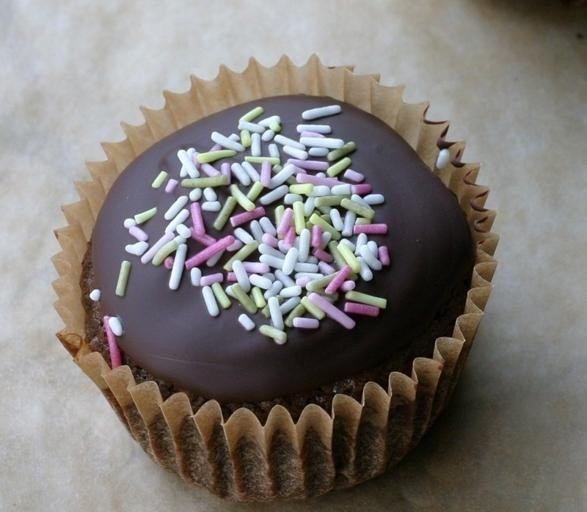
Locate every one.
[52,54,499,502]
